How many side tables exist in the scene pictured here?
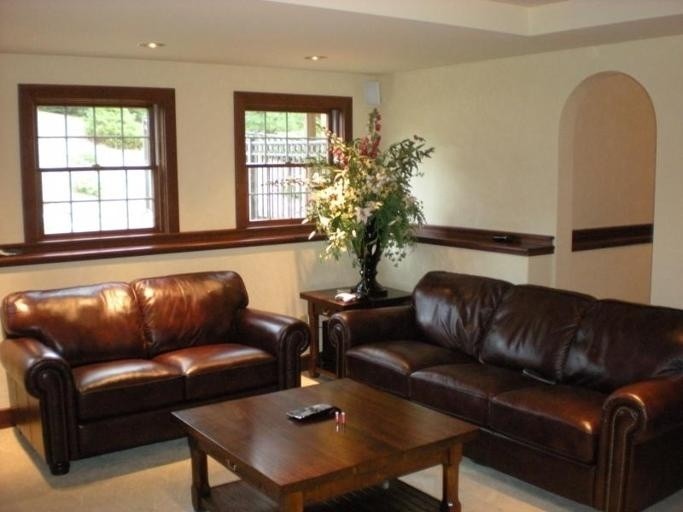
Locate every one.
[300,282,414,378]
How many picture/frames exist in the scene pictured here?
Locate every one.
[351,255,388,297]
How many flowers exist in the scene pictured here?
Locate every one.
[259,108,434,269]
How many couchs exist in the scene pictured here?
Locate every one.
[1,271,311,476]
[326,269,682,512]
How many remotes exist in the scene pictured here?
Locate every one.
[287,404,342,423]
